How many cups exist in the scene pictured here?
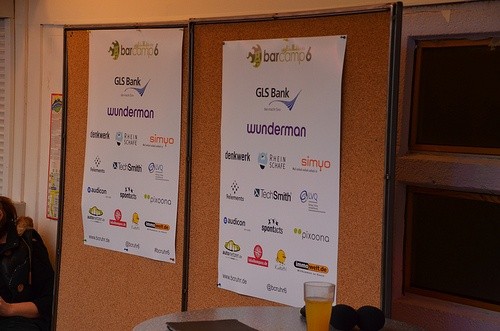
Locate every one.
[304,281,336,331]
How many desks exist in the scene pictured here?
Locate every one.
[132,306,424,331]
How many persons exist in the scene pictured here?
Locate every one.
[0,196,54,331]
